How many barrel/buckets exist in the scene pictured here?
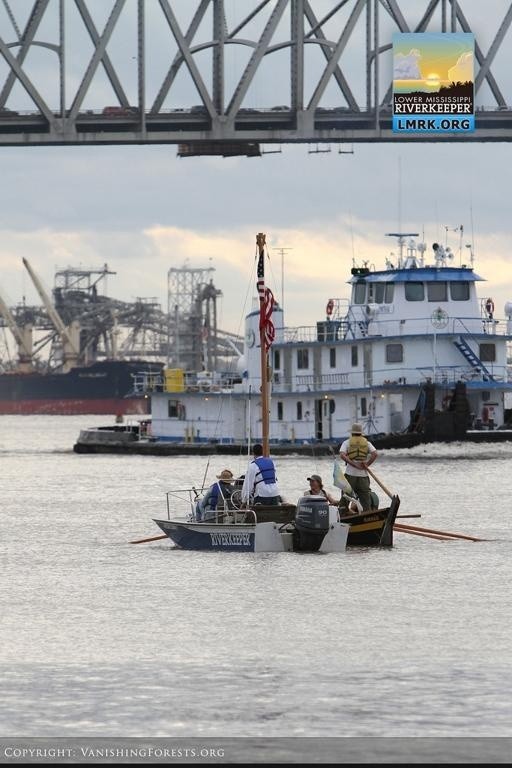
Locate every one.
[329,505,340,523]
[165,369,185,392]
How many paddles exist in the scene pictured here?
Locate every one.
[392,513,498,542]
[129,501,247,545]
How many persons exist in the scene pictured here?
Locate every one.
[304,474,339,504]
[195,469,235,521]
[339,423,378,512]
[240,443,281,510]
[234,474,246,489]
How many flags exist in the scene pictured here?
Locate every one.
[256,250,277,352]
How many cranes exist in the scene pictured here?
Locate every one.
[2,265,244,374]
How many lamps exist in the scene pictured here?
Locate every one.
[367,304,379,316]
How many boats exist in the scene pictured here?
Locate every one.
[150,231,398,550]
[74,224,511,454]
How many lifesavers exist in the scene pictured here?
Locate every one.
[486,299,494,313]
[326,299,335,315]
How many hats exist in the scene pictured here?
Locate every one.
[347,423,365,434]
[307,476,322,483]
[216,470,233,484]
